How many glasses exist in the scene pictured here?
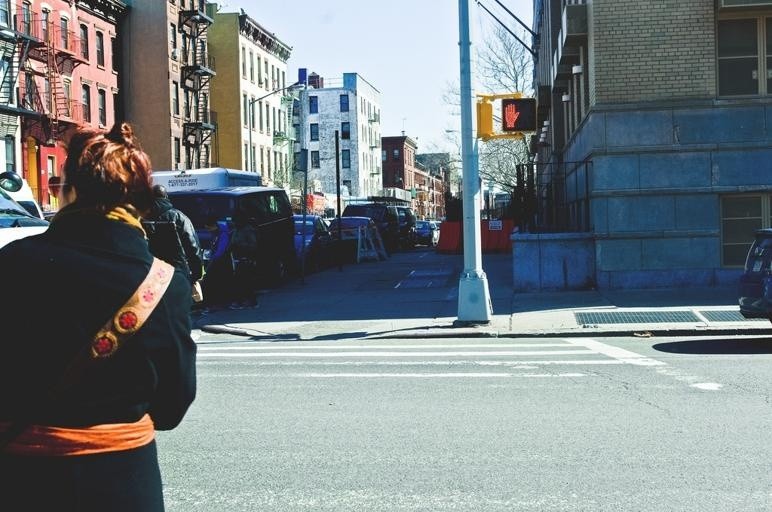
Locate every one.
[48,176,69,198]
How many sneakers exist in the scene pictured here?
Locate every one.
[228,303,259,309]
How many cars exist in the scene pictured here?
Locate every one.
[738,228,772,319]
[294,200,441,259]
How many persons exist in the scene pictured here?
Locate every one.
[226,208,260,311]
[203,214,236,311]
[140,184,204,285]
[0,126,199,512]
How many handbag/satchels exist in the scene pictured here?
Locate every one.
[191,281,203,303]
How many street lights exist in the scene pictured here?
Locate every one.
[248,84,305,172]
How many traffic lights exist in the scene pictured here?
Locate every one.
[476,92,537,141]
